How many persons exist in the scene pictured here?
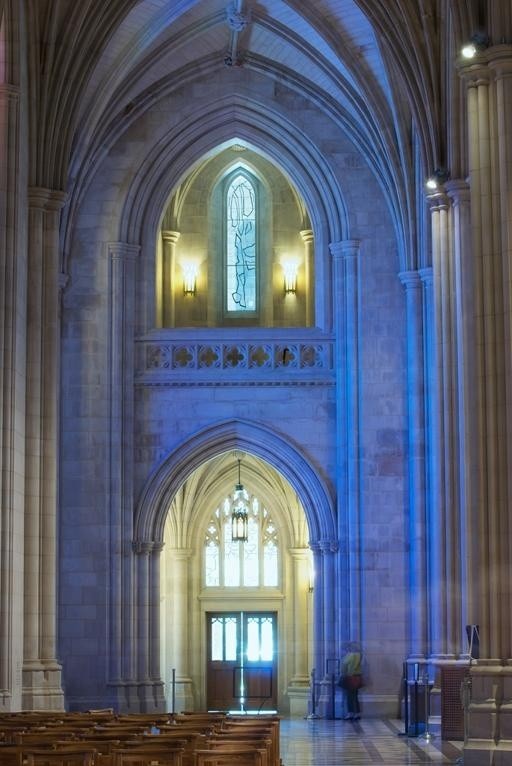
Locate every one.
[336,641,363,720]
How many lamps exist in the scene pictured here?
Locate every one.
[183,269,195,294]
[282,266,298,294]
[425,166,451,190]
[461,29,491,61]
[229,451,251,551]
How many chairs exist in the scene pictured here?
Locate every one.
[0,705,289,766]
